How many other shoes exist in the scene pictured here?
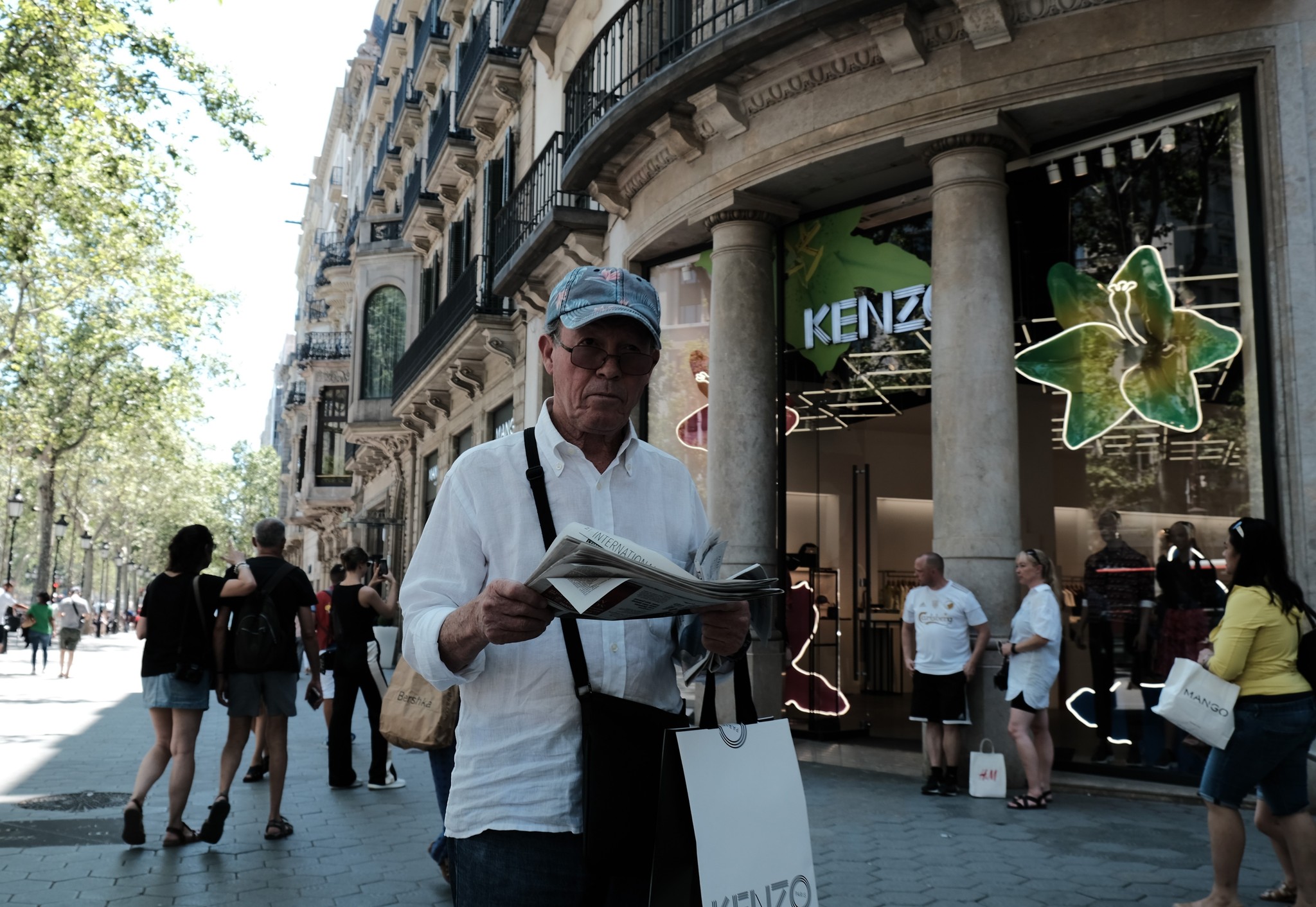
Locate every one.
[921,765,945,794]
[942,766,962,796]
[326,733,356,747]
[367,776,407,790]
[59,673,69,679]
[327,777,363,790]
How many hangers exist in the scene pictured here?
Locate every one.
[885,576,920,591]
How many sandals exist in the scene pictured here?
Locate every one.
[200,794,231,844]
[122,798,146,845]
[1258,882,1297,904]
[243,764,263,782]
[163,821,201,846]
[264,815,294,840]
[1173,892,1242,907]
[1013,789,1054,803]
[261,755,270,775]
[1007,794,1048,810]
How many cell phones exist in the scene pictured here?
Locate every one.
[379,559,387,579]
[308,688,319,711]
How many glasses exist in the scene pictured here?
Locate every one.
[1228,520,1247,540]
[553,333,660,376]
[1025,549,1042,565]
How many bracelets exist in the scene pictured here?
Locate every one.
[1012,642,1018,656]
[233,561,246,574]
[216,671,222,674]
[1203,654,1210,665]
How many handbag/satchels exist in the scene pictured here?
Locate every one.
[379,655,460,751]
[324,648,337,670]
[575,688,697,861]
[79,616,86,629]
[994,661,1009,691]
[177,615,231,690]
[1150,658,1241,750]
[661,635,819,907]
[968,738,1006,799]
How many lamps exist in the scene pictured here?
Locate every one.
[1102,143,1116,167]
[1130,135,1145,159]
[1047,160,1062,184]
[1073,152,1087,177]
[1161,125,1176,152]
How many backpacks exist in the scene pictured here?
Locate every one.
[1295,600,1316,692]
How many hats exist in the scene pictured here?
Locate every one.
[547,265,662,351]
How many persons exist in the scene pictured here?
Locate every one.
[394,265,751,906]
[1076,509,1219,769]
[121,265,751,907]
[25,592,56,674]
[121,523,258,848]
[1172,518,1316,907]
[0,582,29,652]
[998,549,1063,810]
[56,586,90,679]
[902,552,990,797]
